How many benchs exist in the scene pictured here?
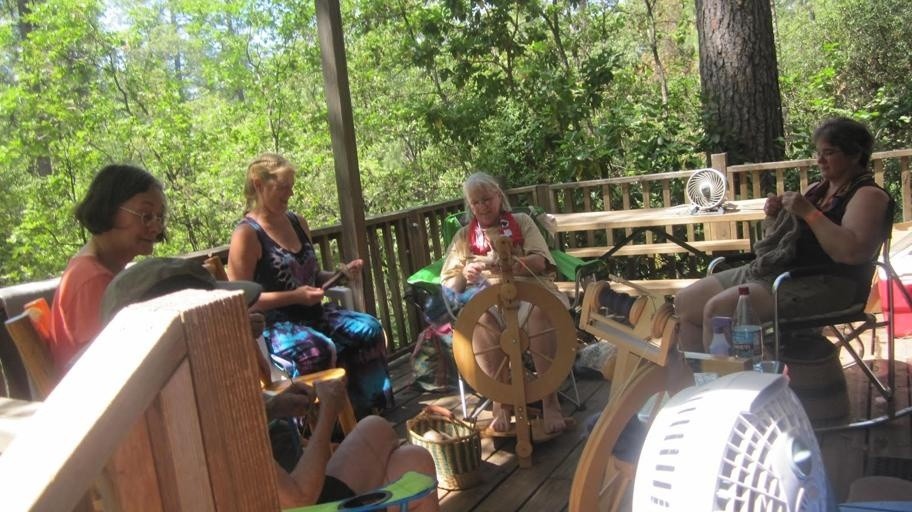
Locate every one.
[547,276,702,298]
[560,238,752,276]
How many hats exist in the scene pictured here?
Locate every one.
[100,257,262,326]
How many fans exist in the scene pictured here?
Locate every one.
[624,363,832,512]
[685,166,728,214]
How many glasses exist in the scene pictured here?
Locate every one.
[117,206,170,228]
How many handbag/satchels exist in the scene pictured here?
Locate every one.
[406,406,481,490]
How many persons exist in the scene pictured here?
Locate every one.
[94,259,437,512]
[440,173,569,434]
[46,165,168,366]
[670,114,895,398]
[228,153,393,419]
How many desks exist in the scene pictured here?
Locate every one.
[548,196,768,273]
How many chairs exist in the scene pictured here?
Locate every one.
[701,187,911,437]
[279,471,437,512]
[432,204,586,428]
[2,294,358,459]
[203,247,400,409]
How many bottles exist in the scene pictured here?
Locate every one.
[730,286,765,365]
[709,325,731,357]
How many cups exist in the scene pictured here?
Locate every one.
[751,360,780,375]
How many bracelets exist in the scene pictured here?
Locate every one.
[806,211,825,222]
[519,257,527,273]
